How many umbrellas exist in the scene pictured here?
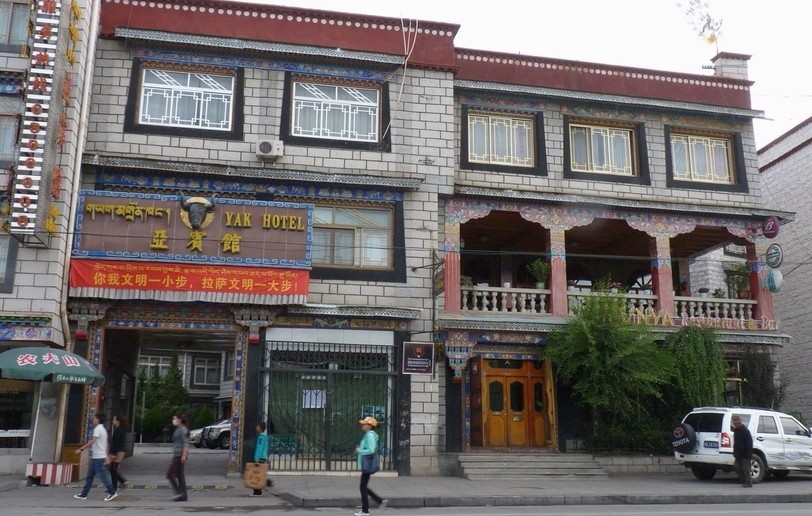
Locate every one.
[0,346,106,462]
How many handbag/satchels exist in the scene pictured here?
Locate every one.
[244,462,268,489]
[362,447,379,474]
[110,451,125,463]
[166,464,177,479]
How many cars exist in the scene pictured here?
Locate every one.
[189,420,229,448]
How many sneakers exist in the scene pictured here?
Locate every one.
[104,492,117,501]
[379,499,388,513]
[249,493,262,497]
[74,494,87,499]
[355,510,369,515]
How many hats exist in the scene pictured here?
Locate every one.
[359,417,377,427]
[731,416,743,423]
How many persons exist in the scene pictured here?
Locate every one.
[250,422,275,496]
[108,415,130,491]
[732,415,753,488]
[166,413,190,502]
[72,413,118,502]
[354,416,387,516]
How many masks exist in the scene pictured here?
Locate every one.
[172,419,180,426]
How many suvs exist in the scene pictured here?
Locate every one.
[201,418,232,450]
[671,406,812,484]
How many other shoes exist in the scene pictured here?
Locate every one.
[173,494,188,502]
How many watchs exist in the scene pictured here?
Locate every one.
[80,447,84,451]
